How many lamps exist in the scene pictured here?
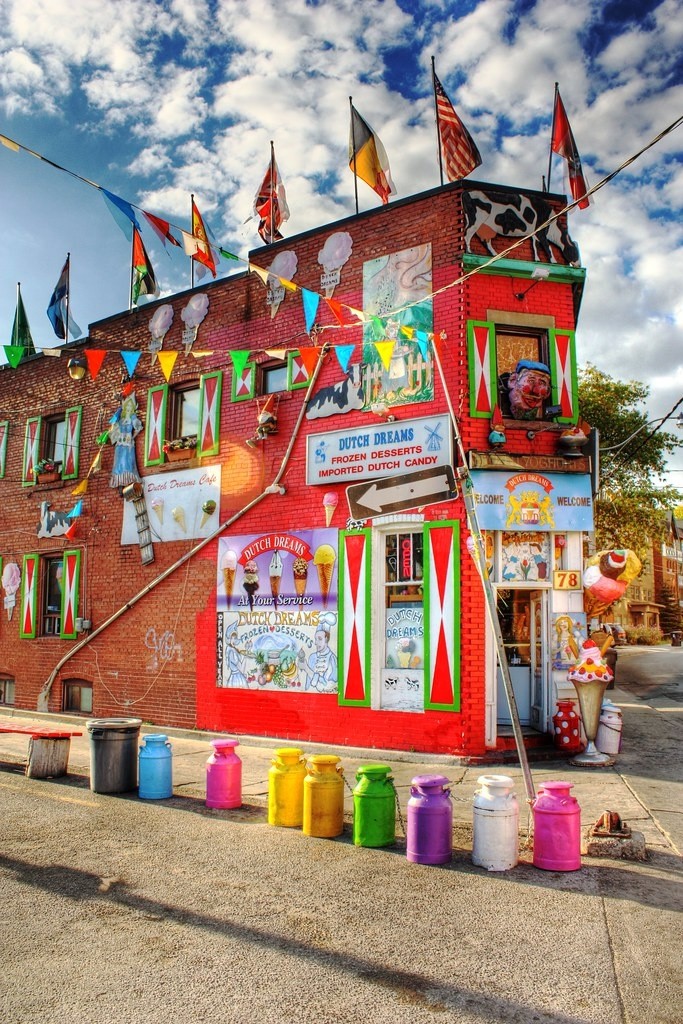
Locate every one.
[372,402,394,423]
[517,267,549,301]
[529,405,563,441]
[67,356,85,379]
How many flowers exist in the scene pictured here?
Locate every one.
[163,437,196,452]
[31,459,61,477]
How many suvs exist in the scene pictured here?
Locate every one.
[607,623,629,646]
[587,623,615,648]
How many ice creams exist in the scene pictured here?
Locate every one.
[151,495,216,532]
[221,545,336,610]
[567,635,614,741]
[323,492,338,528]
[582,547,641,621]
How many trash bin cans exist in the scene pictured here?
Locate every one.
[86,717,143,793]
[671,631,682,646]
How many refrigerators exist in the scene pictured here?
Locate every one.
[497,665,541,727]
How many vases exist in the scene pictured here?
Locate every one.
[165,449,194,459]
[39,472,57,483]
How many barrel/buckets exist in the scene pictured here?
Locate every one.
[353,765,395,847]
[405,774,453,865]
[205,739,243,808]
[533,781,582,871]
[303,755,344,837]
[268,748,307,827]
[552,702,580,750]
[472,775,519,872]
[593,705,622,755]
[139,734,173,799]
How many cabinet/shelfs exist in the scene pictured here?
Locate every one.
[498,598,531,616]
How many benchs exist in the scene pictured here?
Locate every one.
[0,722,82,779]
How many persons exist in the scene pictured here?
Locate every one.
[497,360,551,421]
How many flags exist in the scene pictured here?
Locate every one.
[46,253,82,340]
[347,96,396,210]
[130,223,161,305]
[8,281,36,358]
[252,139,290,245]
[670,514,678,540]
[549,83,594,214]
[190,194,217,279]
[432,56,483,183]
